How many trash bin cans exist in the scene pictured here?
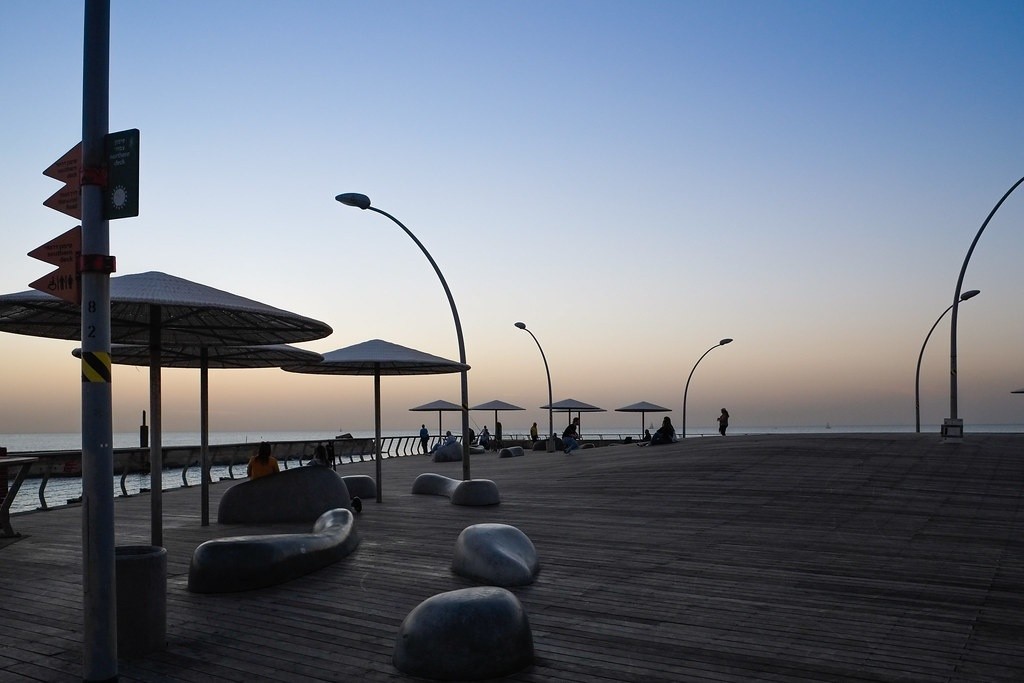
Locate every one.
[546,438,555,453]
[940,418,964,444]
[114,544,167,656]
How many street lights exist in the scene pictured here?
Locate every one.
[914,288,980,433]
[335,192,472,481]
[514,321,553,437]
[682,338,733,437]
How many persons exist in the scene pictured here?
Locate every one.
[640,420,673,446]
[717,408,730,437]
[306,445,333,468]
[530,423,539,447]
[419,423,429,454]
[247,441,280,481]
[477,425,489,438]
[468,427,476,445]
[429,431,456,456]
[642,429,651,442]
[561,417,581,455]
[481,429,489,446]
[663,416,679,444]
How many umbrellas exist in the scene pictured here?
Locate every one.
[468,399,527,446]
[552,407,608,441]
[539,399,601,434]
[280,338,471,504]
[72,336,325,529]
[408,399,466,445]
[1,272,335,550]
[614,401,672,440]
